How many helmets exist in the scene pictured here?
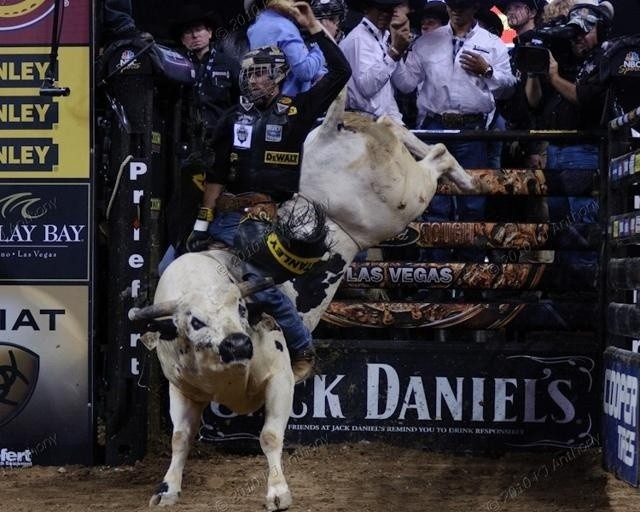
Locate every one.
[238,47,292,103]
[310,0,346,22]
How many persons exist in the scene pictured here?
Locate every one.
[98,1,639,385]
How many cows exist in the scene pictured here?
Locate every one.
[128,0,492,512]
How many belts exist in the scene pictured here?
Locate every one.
[215,192,275,212]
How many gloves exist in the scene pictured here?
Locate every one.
[186,230,211,253]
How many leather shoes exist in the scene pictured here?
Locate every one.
[291,347,316,384]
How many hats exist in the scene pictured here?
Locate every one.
[406,0,549,38]
